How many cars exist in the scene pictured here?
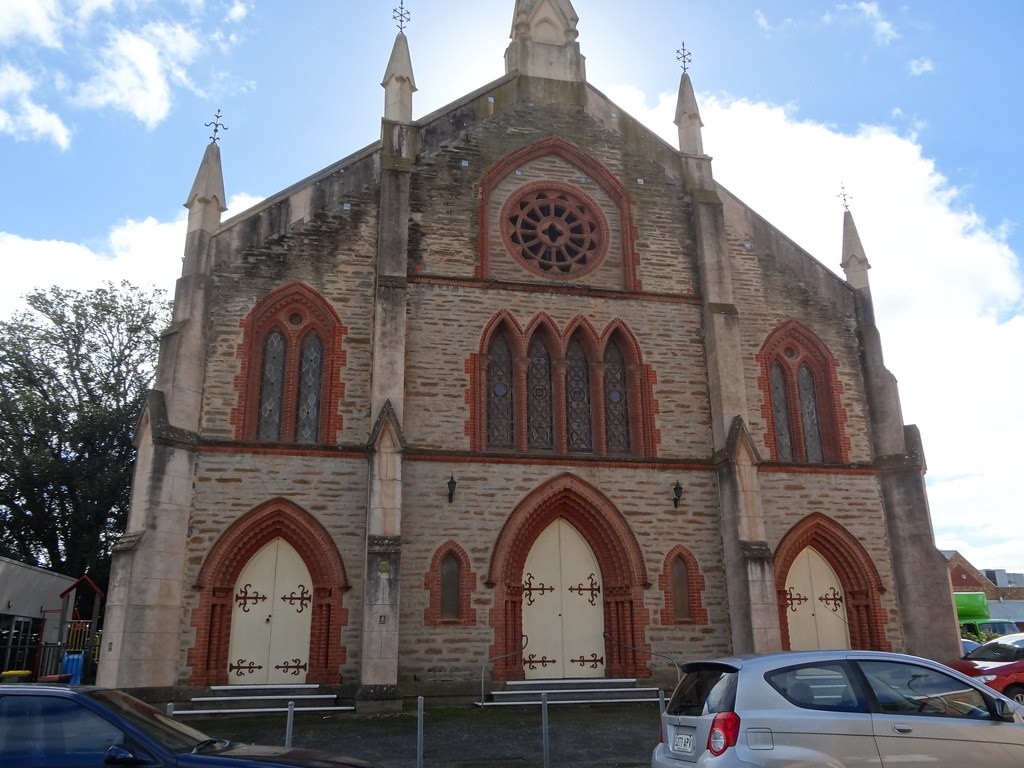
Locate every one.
[0,682,380,768]
[646,648,1024,768]
[946,629,1024,707]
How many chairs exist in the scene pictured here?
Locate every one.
[842,686,852,700]
[790,683,815,703]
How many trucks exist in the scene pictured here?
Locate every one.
[953,591,1021,635]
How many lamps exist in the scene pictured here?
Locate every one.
[446,472,457,503]
[673,479,683,509]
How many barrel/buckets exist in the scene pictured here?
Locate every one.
[61,651,83,684]
